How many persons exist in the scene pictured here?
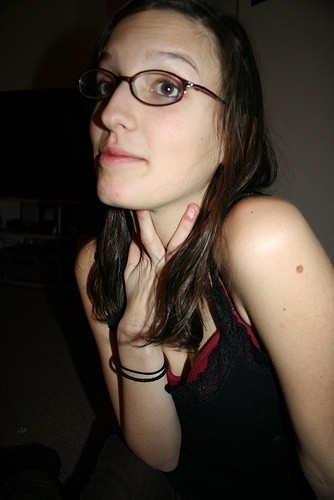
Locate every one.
[71,1,333,499]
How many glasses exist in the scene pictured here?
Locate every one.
[77,68,229,106]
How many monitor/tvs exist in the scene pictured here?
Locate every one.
[0,86,100,205]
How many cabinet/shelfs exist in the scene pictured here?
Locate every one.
[0,195,104,293]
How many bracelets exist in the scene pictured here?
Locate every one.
[113,352,167,382]
[118,358,166,375]
